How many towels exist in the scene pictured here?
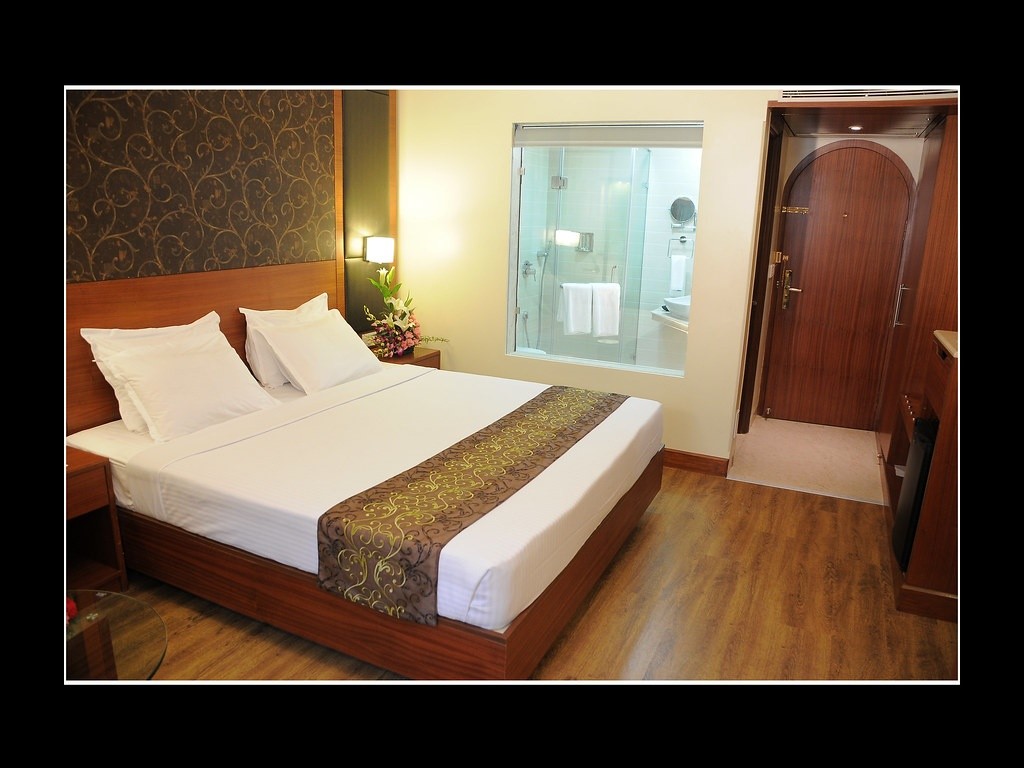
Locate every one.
[554,230,594,252]
[557,283,620,337]
[669,255,686,291]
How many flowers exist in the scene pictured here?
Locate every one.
[360,266,450,358]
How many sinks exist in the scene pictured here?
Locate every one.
[664,294,691,316]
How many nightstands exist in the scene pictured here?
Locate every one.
[371,347,440,369]
[67,446,127,610]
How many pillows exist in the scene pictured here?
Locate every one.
[256,308,384,395]
[238,293,330,388]
[79,311,221,433]
[92,319,275,442]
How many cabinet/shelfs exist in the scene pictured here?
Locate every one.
[878,112,958,624]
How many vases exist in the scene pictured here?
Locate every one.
[393,344,414,358]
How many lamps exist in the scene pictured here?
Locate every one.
[363,235,395,265]
[555,229,594,252]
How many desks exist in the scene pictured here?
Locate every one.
[66,590,168,680]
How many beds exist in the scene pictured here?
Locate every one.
[66,261,666,681]
